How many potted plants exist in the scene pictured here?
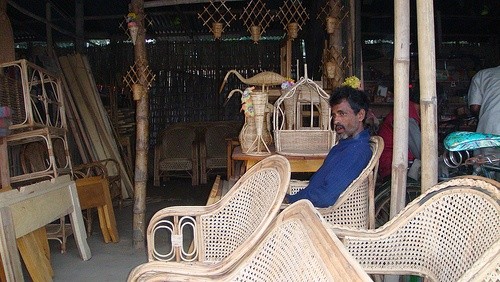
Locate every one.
[373,89,385,104]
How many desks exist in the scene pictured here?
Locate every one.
[232,139,329,183]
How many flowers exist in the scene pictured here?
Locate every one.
[281,77,295,91]
[127,13,139,45]
[341,75,361,89]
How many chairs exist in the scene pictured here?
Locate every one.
[199,123,242,185]
[138,199,374,282]
[280,136,384,232]
[153,126,199,187]
[21,138,123,254]
[322,174,500,282]
[123,155,291,281]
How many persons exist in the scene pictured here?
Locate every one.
[467,66,500,166]
[378,82,423,183]
[281,84,374,209]
